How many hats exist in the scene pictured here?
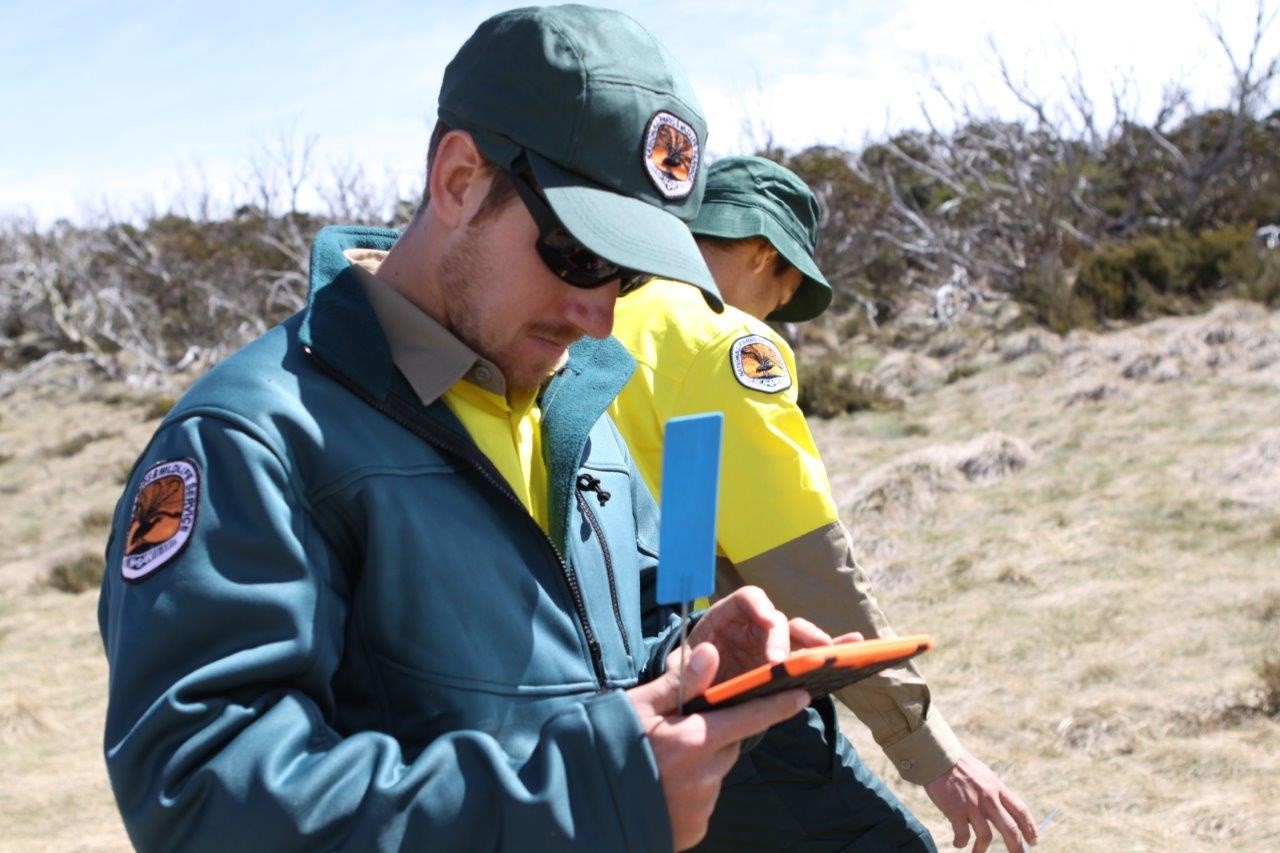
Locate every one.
[685,155,833,323]
[436,3,726,316]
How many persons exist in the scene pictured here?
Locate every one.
[95,0,861,853]
[594,151,1040,853]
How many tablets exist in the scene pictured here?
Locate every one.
[681,633,929,723]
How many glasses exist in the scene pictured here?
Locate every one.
[501,163,659,298]
